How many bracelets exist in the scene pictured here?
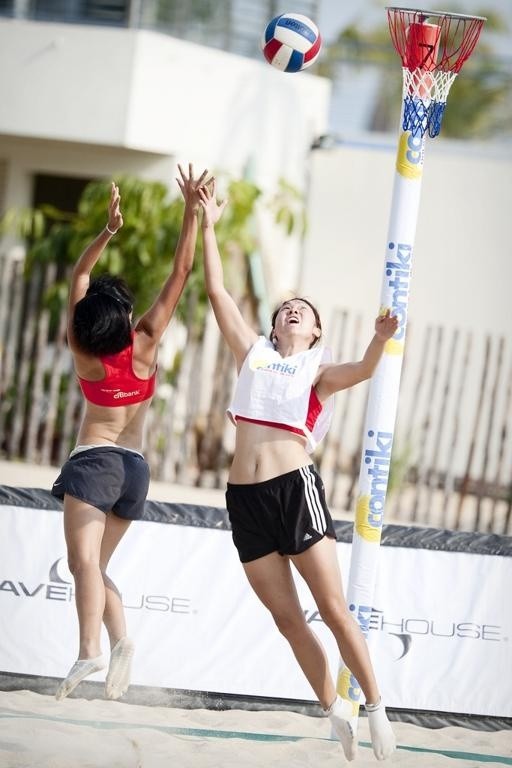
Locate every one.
[106,224,119,235]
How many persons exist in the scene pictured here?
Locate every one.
[198,179,402,762]
[53,161,218,700]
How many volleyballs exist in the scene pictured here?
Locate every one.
[261,11,322,73]
